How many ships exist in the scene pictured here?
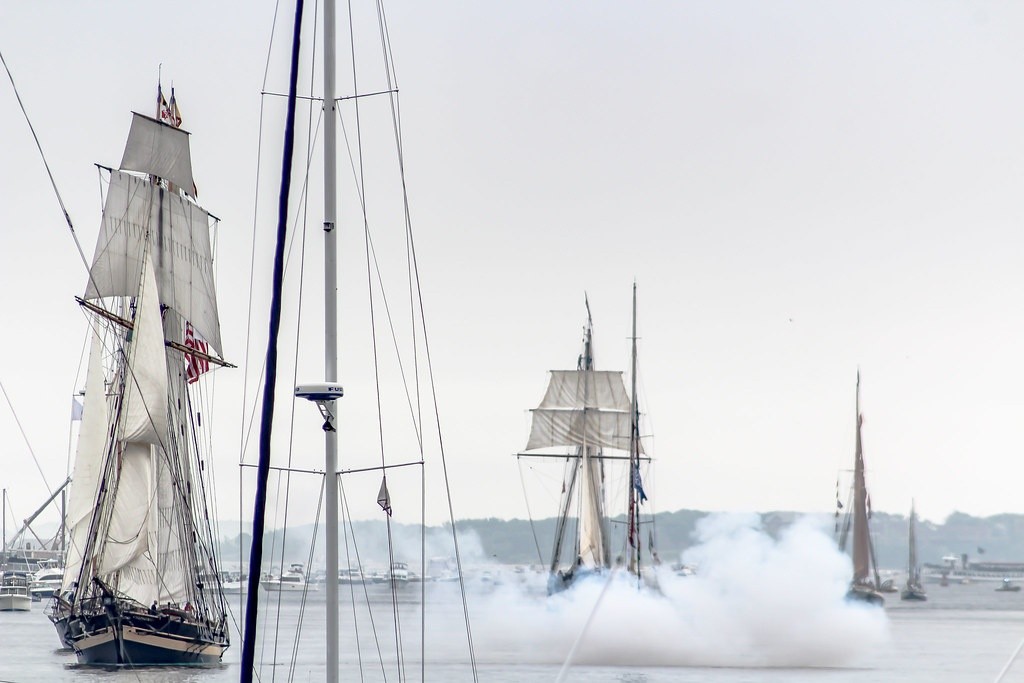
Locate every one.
[923,553,1024,585]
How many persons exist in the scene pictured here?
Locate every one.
[185,601,195,616]
[577,354,592,370]
[151,600,158,615]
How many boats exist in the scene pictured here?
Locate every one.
[994,577,1020,592]
[3,476,703,621]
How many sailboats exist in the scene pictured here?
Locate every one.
[829,372,902,611]
[506,279,668,611]
[899,497,929,601]
[44,65,241,669]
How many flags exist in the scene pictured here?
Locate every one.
[157,84,178,128]
[633,462,648,505]
[168,87,182,127]
[183,321,210,385]
